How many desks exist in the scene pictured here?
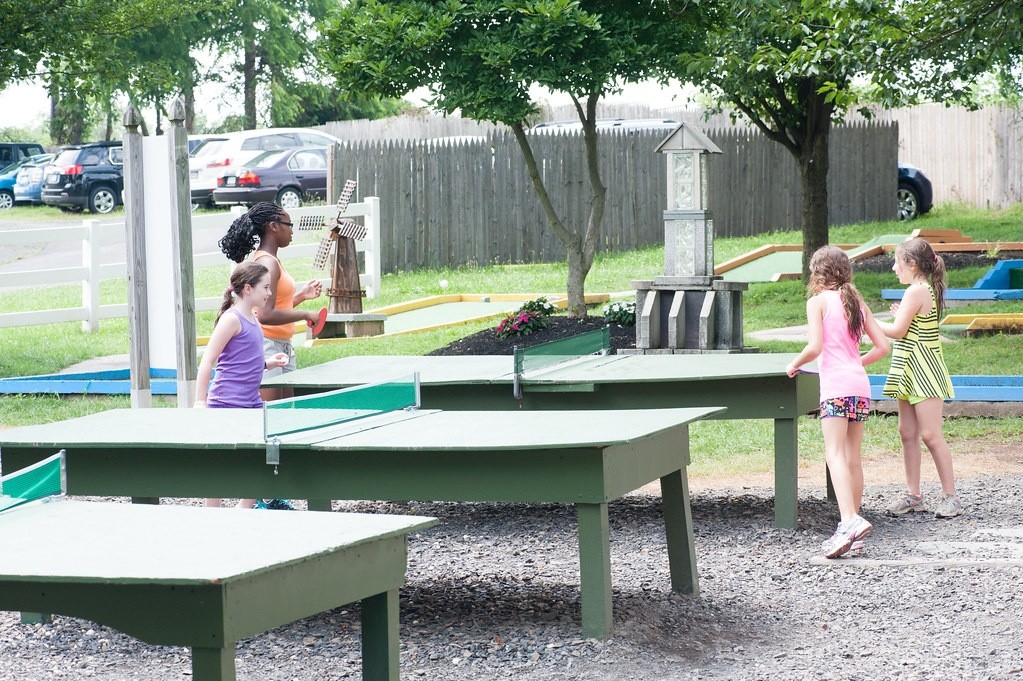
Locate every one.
[2,370,730,639]
[258,323,822,531]
[0,448,440,680]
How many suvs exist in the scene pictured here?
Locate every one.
[41,141,125,215]
[0,142,47,172]
[189,128,353,208]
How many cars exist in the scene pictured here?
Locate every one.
[0,158,31,209]
[213,146,329,210]
[14,155,54,201]
[898,162,934,223]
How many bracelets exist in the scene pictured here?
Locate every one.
[263,360,267,369]
[790,362,800,371]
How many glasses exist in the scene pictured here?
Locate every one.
[281,221,293,228]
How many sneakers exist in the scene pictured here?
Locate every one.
[886,492,927,517]
[823,514,872,558]
[936,492,964,518]
[257,498,298,511]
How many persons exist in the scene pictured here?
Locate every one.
[785,246,891,559]
[192,262,288,509]
[871,238,963,520]
[218,201,323,402]
